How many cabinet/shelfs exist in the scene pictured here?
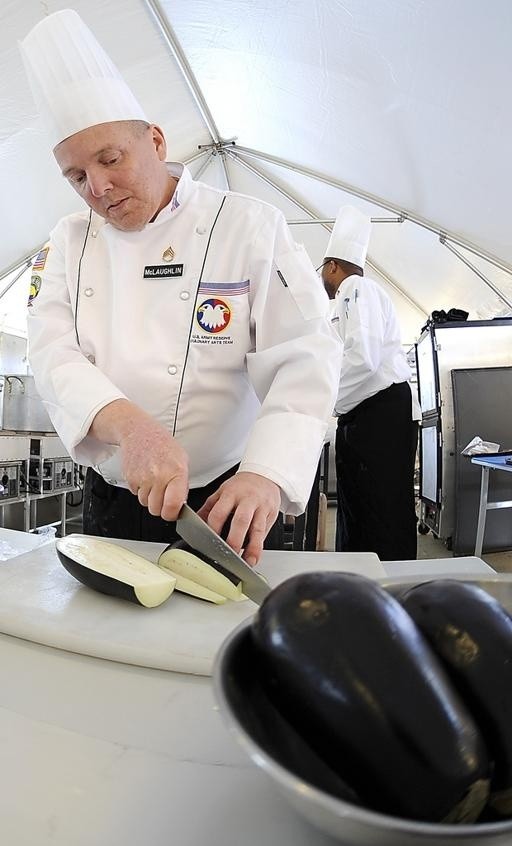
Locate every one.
[0,484,84,537]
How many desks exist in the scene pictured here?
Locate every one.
[470,452,512,557]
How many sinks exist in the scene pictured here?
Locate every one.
[0,526,59,562]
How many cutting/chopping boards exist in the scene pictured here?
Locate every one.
[0,532,387,681]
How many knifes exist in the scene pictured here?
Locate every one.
[174,502,272,607]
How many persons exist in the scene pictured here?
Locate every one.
[27,114,344,567]
[318,253,424,562]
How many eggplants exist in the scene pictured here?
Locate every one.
[251,570,494,826]
[397,580,512,820]
[55,532,250,609]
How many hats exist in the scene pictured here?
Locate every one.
[323,205,374,269]
[19,7,155,153]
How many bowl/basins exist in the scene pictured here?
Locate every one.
[213,571,511,845]
